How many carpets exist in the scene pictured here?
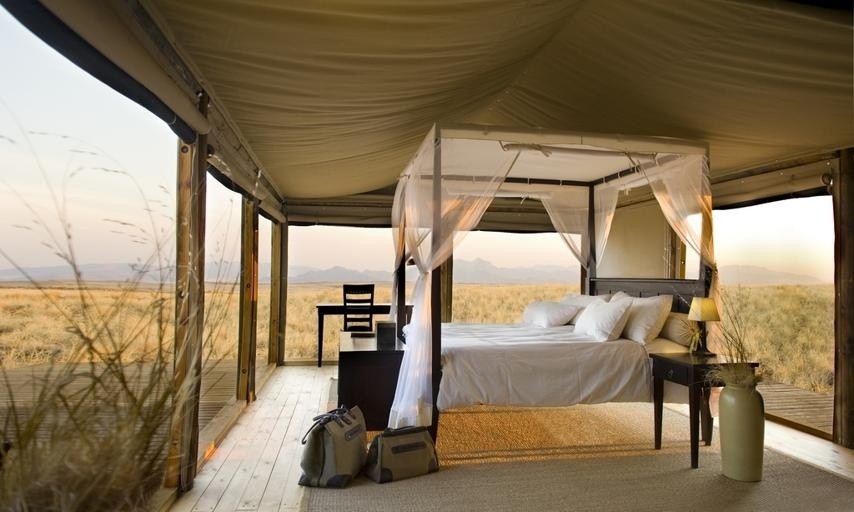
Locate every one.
[299,379,854,512]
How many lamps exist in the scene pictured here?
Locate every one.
[686,296,721,357]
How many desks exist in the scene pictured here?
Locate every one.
[648,353,759,471]
[316,303,414,367]
[338,332,408,432]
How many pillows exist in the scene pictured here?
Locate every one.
[522,290,701,346]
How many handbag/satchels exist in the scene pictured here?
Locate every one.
[365,425,439,485]
[298,405,368,489]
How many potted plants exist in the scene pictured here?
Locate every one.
[670,269,764,482]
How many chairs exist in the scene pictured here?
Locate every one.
[342,283,375,332]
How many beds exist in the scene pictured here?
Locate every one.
[397,119,712,449]
